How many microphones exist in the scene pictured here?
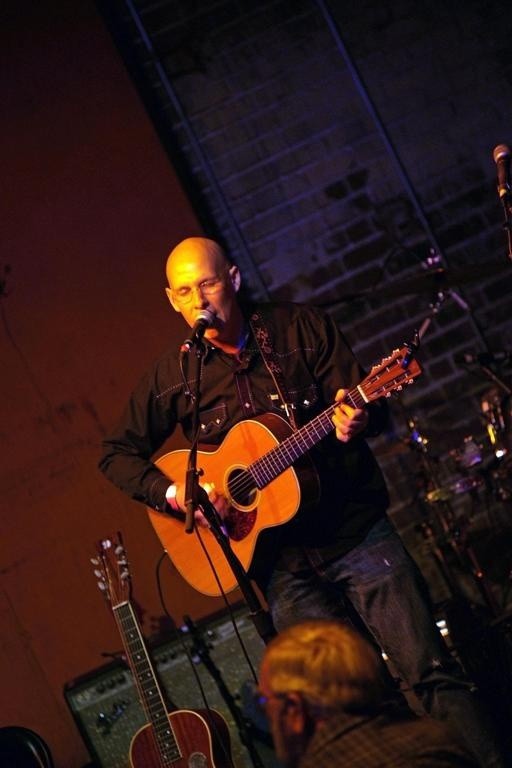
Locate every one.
[391,260,478,295]
[180,310,215,354]
[493,145,510,202]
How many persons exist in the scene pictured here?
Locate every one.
[259,621,477,768]
[96,235,475,723]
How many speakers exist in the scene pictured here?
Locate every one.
[63,600,279,768]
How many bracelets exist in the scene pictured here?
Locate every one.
[165,483,181,512]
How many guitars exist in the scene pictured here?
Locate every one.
[91,537,233,766]
[146,347,422,596]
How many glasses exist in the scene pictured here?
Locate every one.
[172,274,226,306]
